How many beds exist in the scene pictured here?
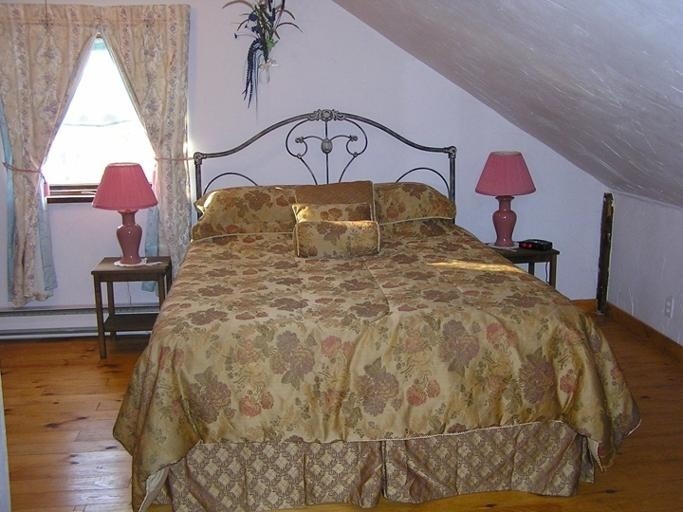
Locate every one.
[113,110,642,511]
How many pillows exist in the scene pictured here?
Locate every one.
[291,218,379,257]
[369,184,459,226]
[192,178,373,240]
[289,200,374,225]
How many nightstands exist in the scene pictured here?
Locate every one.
[488,241,561,286]
[90,258,173,357]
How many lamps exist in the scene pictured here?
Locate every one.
[95,160,170,265]
[475,150,533,245]
